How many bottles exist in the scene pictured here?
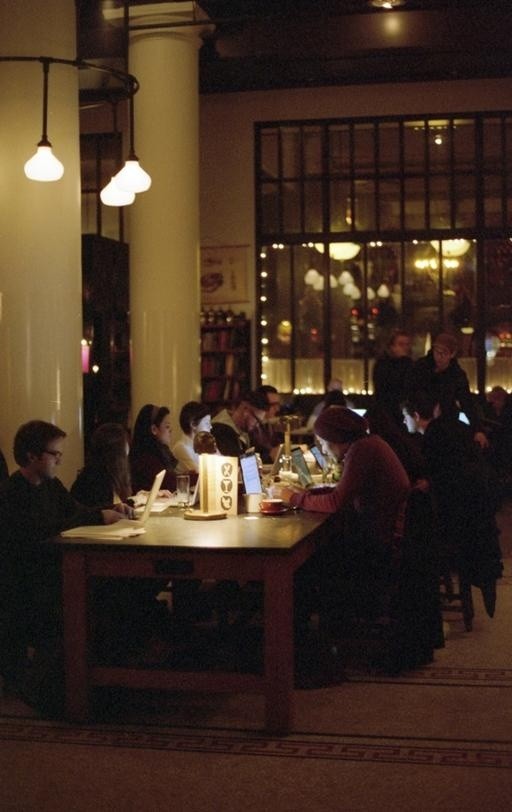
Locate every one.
[280,415,299,430]
[200,304,234,327]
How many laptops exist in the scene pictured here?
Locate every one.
[118,469,166,527]
[238,452,297,510]
[266,443,284,478]
[309,444,331,474]
[291,447,337,490]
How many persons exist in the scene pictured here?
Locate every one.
[131,404,216,616]
[371,335,512,540]
[2,420,137,660]
[278,380,353,429]
[176,387,281,471]
[286,408,412,648]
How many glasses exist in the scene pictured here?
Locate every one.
[45,451,62,459]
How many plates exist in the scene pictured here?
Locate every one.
[258,509,290,514]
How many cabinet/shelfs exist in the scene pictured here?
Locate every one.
[201,314,251,402]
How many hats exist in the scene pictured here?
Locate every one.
[315,406,367,442]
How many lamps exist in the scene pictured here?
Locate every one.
[23,59,65,182]
[99,98,135,208]
[115,83,153,192]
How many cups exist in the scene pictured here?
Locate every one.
[175,474,191,512]
[279,455,291,474]
[258,499,283,511]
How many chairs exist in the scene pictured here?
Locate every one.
[340,477,431,670]
[437,430,491,624]
